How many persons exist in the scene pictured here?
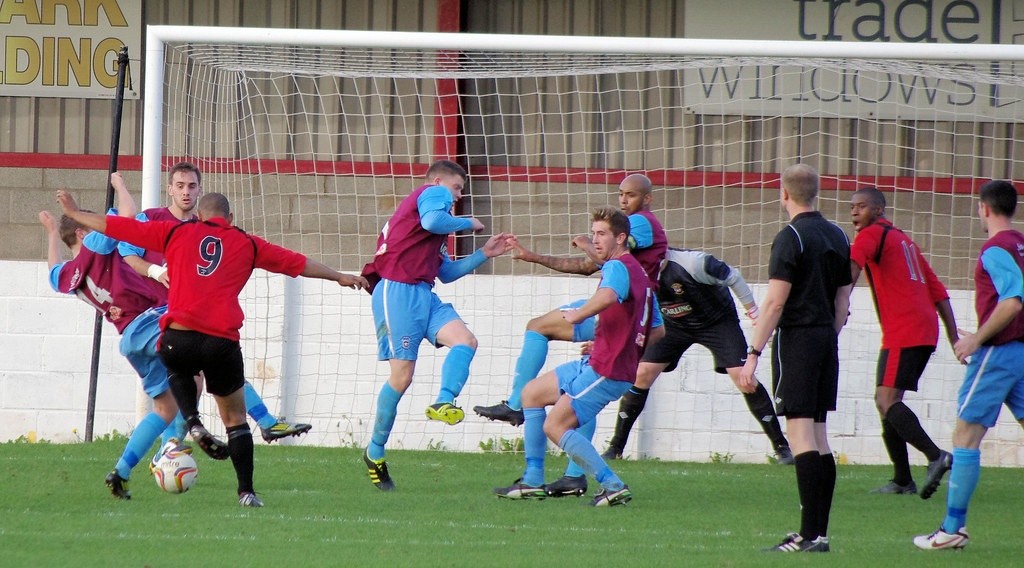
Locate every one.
[360,160,518,493]
[473,176,792,508]
[739,163,851,552]
[843,187,968,499]
[54,187,368,507]
[38,162,313,502]
[913,180,1024,553]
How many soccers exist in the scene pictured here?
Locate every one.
[154,452,198,494]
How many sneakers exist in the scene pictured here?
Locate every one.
[774,445,794,465]
[104,468,131,499]
[190,425,229,460]
[914,522,969,550]
[869,478,917,494]
[150,438,193,475]
[493,477,546,501]
[590,485,632,506]
[919,450,953,499]
[363,444,395,490]
[473,400,524,427]
[425,400,466,425]
[542,475,588,497]
[261,418,313,442]
[761,533,830,553]
[600,445,623,459]
[239,490,264,507]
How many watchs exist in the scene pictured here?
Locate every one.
[746,346,762,357]
